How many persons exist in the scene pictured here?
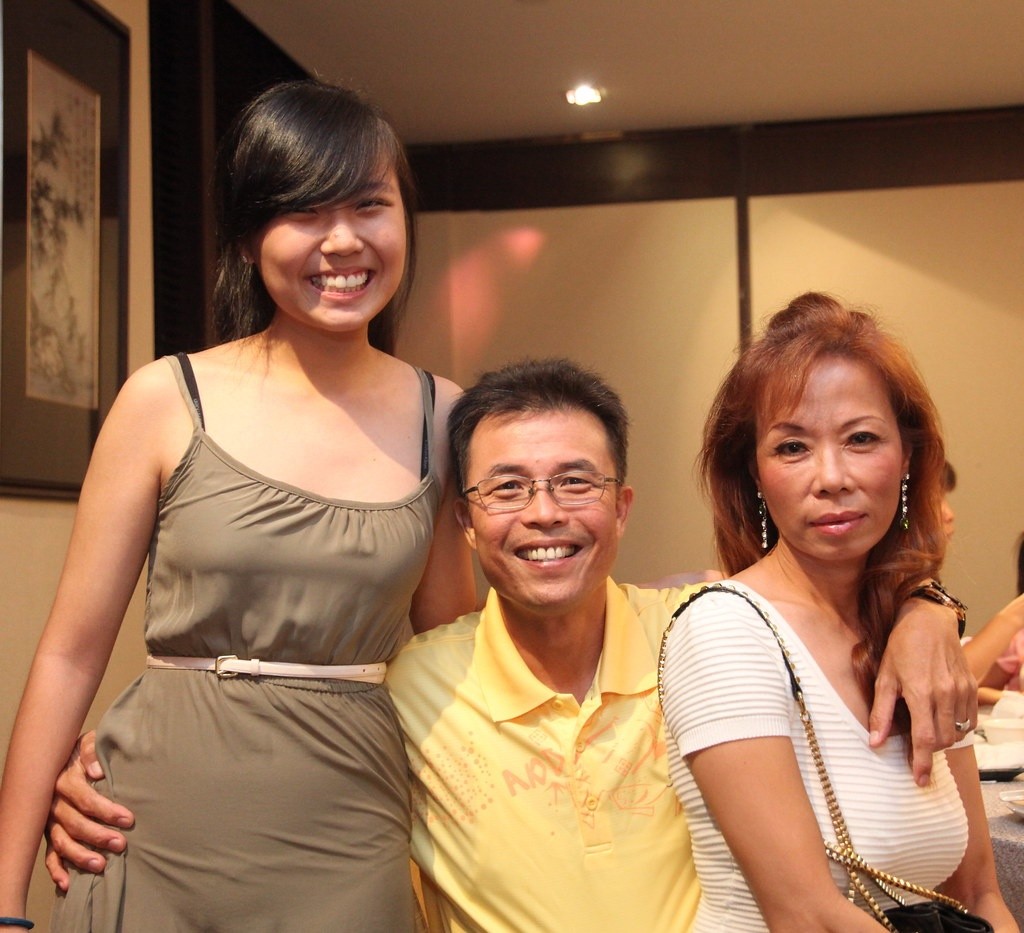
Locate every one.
[656,292,1024,933]
[41,357,978,933]
[917,461,1024,688]
[978,537,1024,705]
[0,80,478,933]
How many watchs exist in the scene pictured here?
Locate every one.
[911,580,967,640]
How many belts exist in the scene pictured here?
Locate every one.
[145,655,386,684]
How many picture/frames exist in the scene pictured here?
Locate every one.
[1,1,137,506]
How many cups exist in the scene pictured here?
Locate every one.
[983,718,1024,744]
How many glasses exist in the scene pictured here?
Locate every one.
[461,471,623,512]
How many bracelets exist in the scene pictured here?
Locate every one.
[0,917,35,930]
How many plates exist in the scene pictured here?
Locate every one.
[979,768,1024,782]
[1006,801,1024,817]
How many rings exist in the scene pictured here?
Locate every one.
[956,719,970,731]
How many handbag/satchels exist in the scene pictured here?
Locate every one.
[657,588,995,933]
